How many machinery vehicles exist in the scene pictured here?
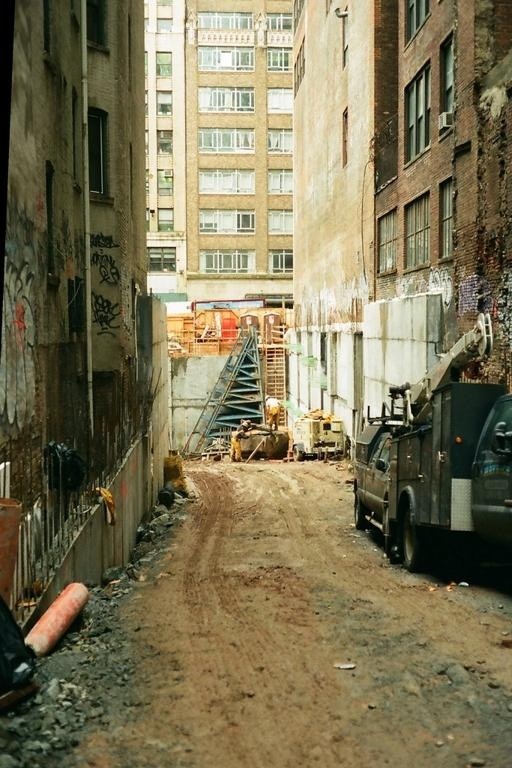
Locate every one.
[349,313,512,578]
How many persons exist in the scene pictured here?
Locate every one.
[264,394,282,432]
[229,425,250,462]
